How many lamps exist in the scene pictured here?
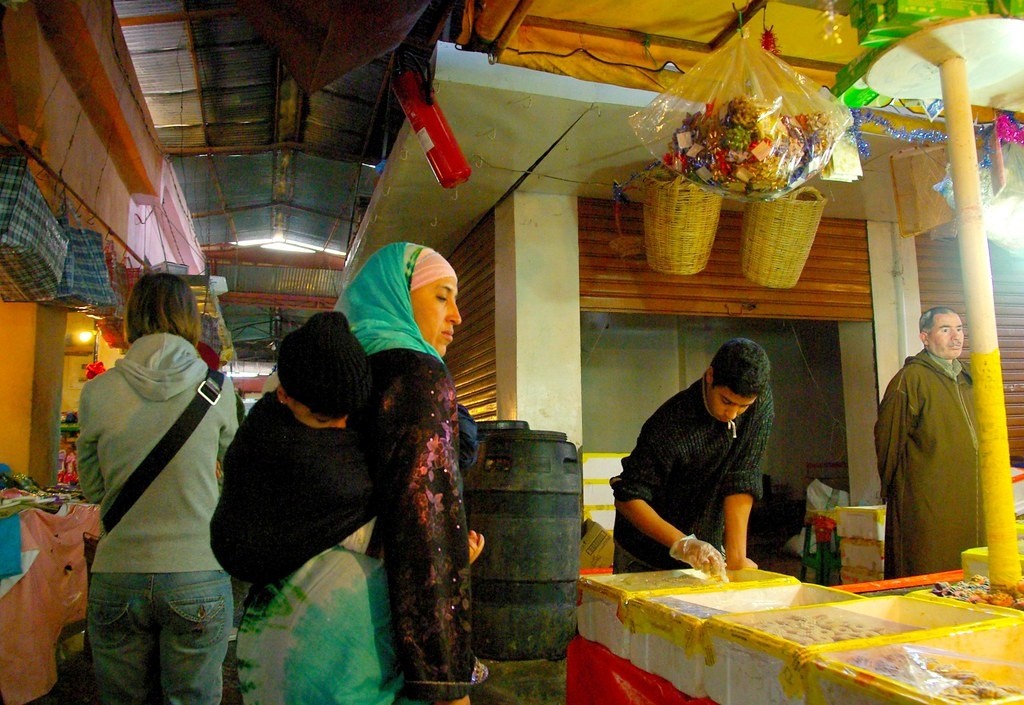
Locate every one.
[209,274,228,296]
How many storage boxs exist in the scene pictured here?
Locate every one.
[581,453,632,569]
[834,504,887,584]
[577,565,1023,705]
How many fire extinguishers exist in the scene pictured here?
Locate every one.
[389,48,472,190]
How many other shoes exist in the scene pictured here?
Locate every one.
[469,534,485,566]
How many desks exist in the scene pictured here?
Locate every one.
[0,492,103,705]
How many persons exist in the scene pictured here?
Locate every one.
[873,306,975,580]
[609,339,774,580]
[75,273,239,705]
[208,242,484,705]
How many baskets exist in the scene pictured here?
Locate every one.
[640,169,723,276]
[742,186,828,290]
